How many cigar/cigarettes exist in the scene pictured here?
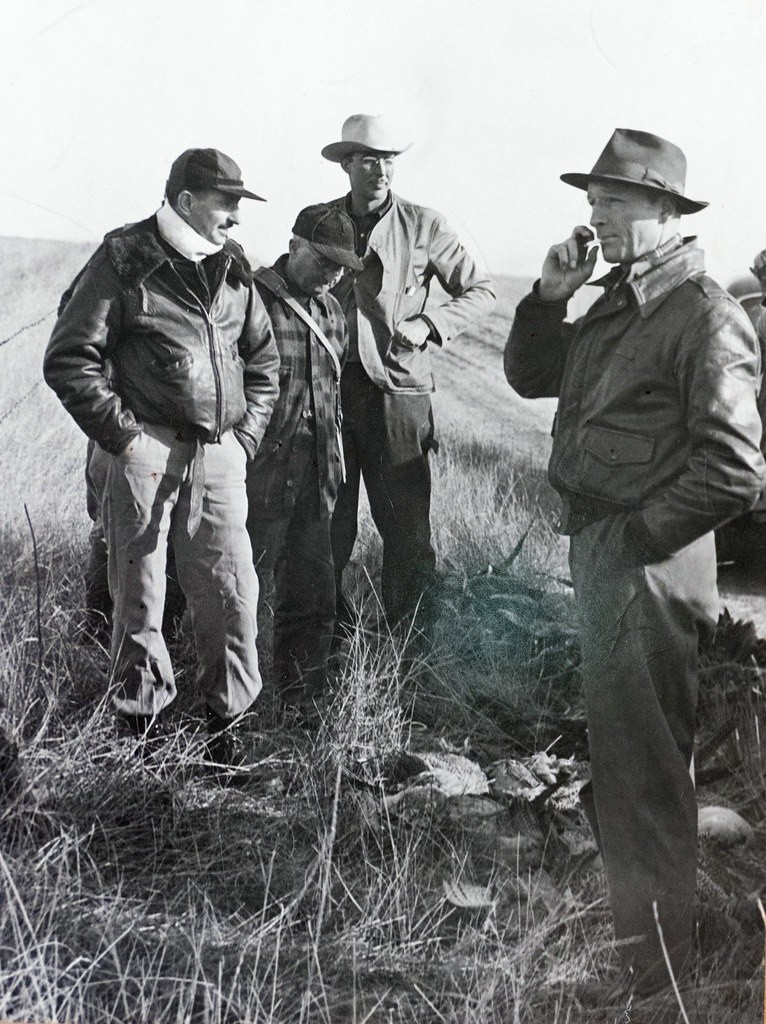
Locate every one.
[583,238,600,248]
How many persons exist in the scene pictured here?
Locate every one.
[43,147,364,780]
[322,114,495,656]
[503,128,766,1007]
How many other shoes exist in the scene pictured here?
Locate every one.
[81,581,110,647]
[578,982,640,1007]
[123,716,162,768]
[202,704,247,787]
[162,576,187,639]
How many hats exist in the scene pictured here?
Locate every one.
[292,204,364,272]
[322,114,413,163]
[563,128,709,214]
[165,148,266,201]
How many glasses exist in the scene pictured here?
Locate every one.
[347,157,396,172]
[301,245,351,276]
